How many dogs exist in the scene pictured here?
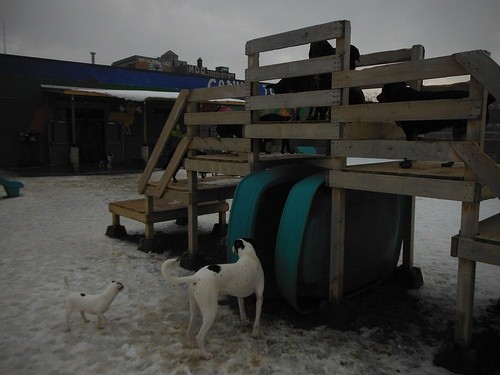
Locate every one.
[160,238,264,359]
[377,82,496,169]
[63,275,124,332]
[264,40,361,123]
